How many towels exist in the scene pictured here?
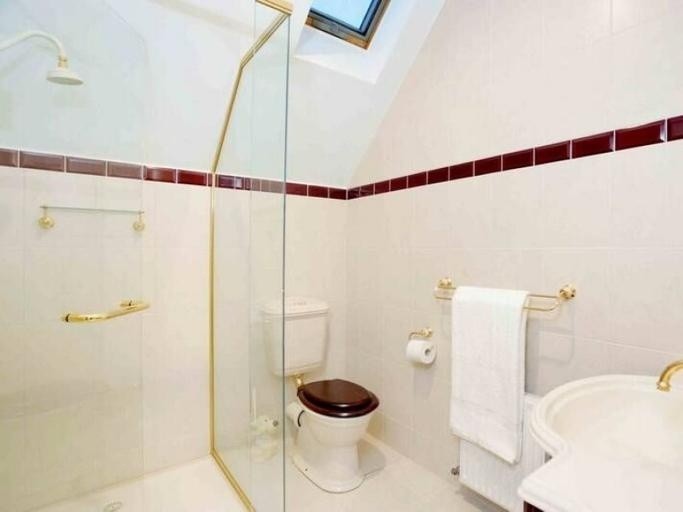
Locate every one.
[448,286,530,466]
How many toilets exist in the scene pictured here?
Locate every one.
[262,300,380,494]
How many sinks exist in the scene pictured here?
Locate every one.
[517,375,683,494]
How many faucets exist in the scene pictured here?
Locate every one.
[656,362,683,392]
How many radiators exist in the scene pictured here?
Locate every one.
[456,388,543,512]
